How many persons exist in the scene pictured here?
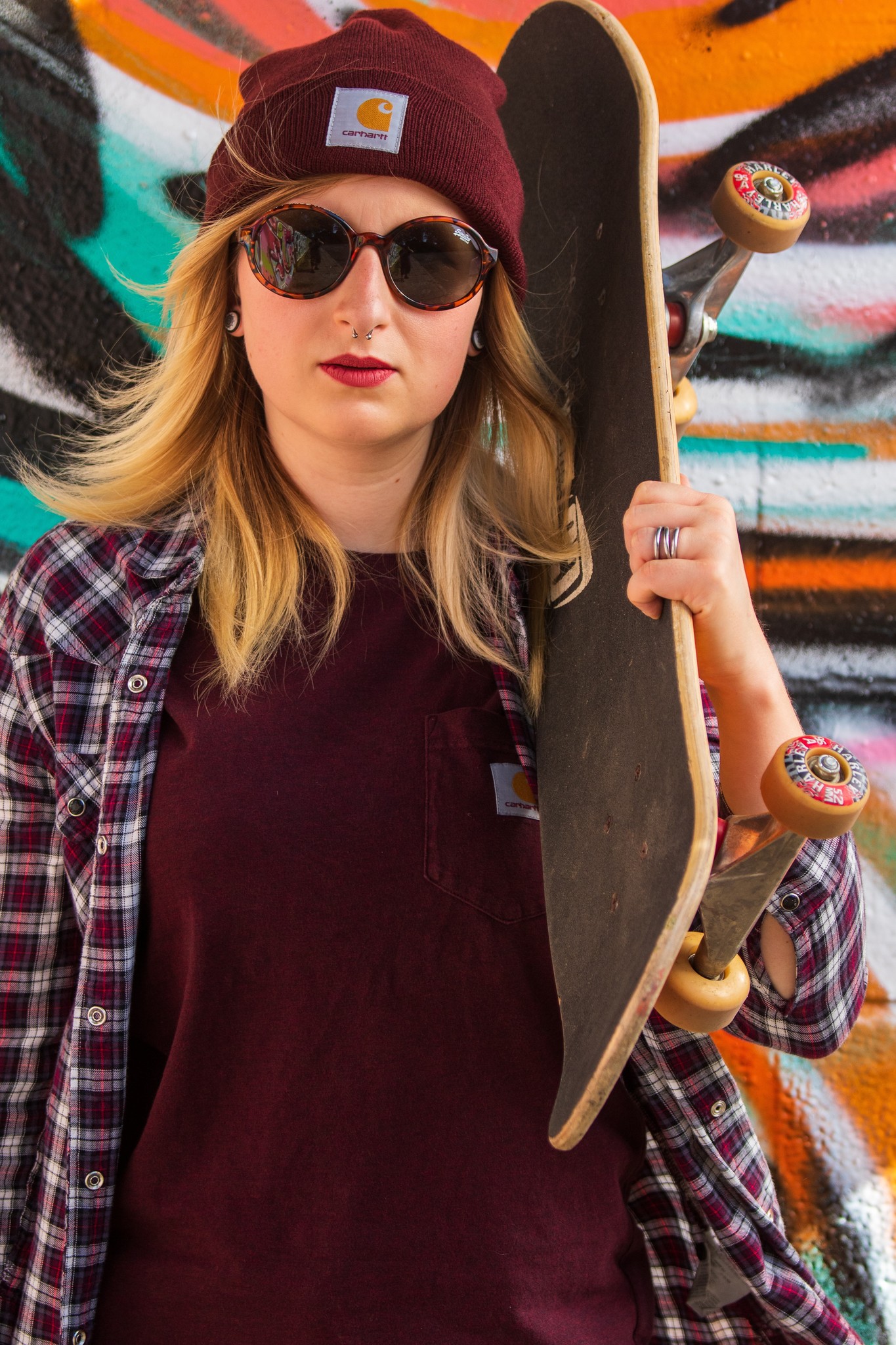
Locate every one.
[0,19,869,1345]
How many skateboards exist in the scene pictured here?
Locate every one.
[493,0,874,1157]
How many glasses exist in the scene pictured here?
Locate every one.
[233,204,498,311]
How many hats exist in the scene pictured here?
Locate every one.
[195,10,526,305]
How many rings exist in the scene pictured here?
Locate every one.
[653,525,679,560]
